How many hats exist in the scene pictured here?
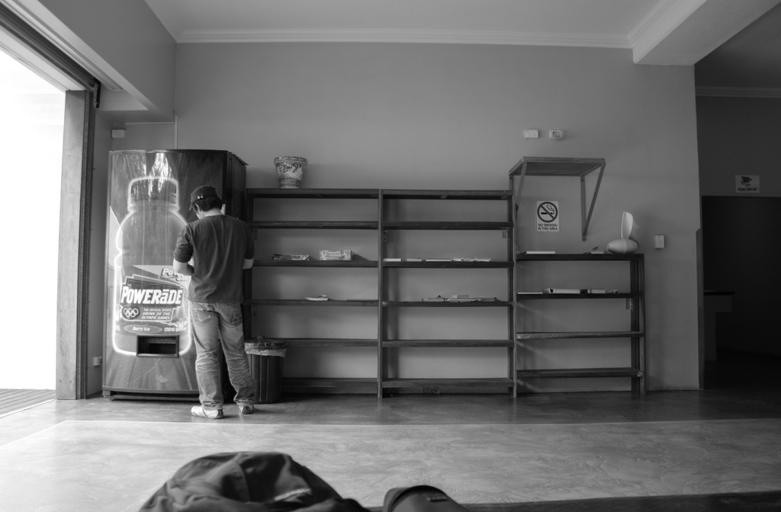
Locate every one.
[189,185,217,211]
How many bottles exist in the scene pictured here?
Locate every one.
[111,173,195,360]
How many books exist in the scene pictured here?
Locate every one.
[523,249,556,255]
[583,287,621,294]
[542,286,587,294]
[518,289,544,294]
[384,257,494,263]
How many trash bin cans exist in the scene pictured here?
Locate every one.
[243,339,288,404]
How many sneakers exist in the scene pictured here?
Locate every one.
[192,405,223,419]
[240,403,254,414]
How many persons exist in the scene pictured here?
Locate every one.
[171,185,259,420]
